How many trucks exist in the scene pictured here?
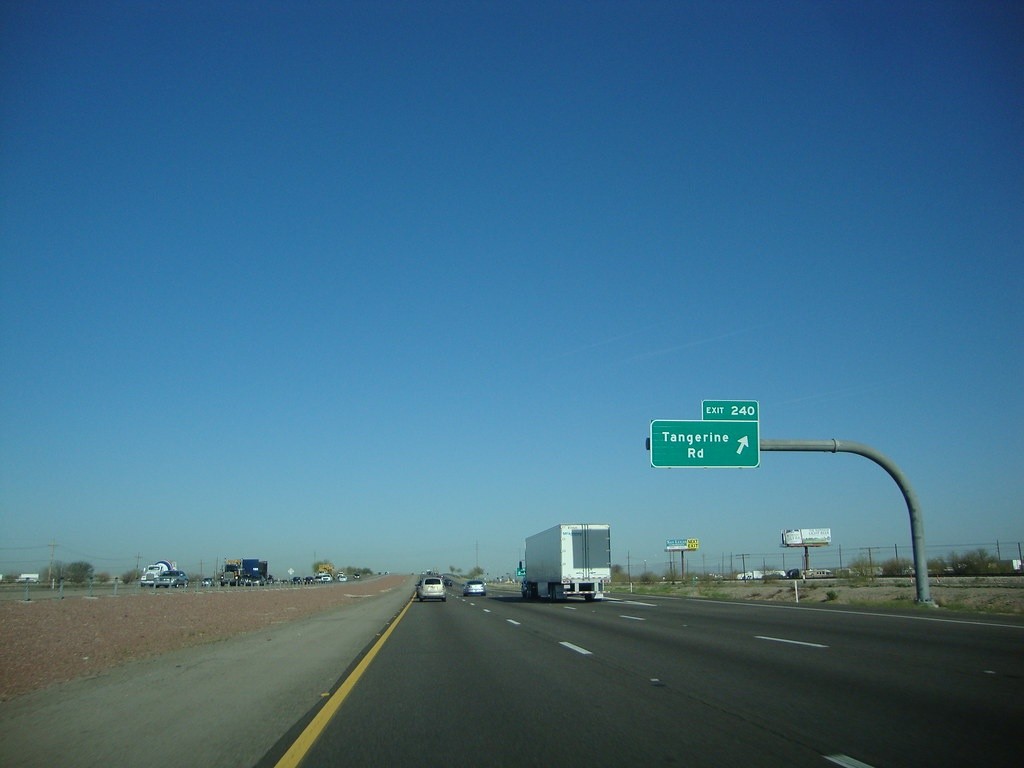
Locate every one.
[521,522,611,602]
[13,574,40,584]
[221,557,267,587]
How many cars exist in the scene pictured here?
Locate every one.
[461,580,487,596]
[427,570,453,587]
[417,577,446,603]
[267,572,362,584]
[154,570,190,589]
[202,577,216,588]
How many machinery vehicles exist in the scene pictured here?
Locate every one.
[141,560,178,588]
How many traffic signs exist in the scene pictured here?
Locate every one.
[648,419,760,470]
